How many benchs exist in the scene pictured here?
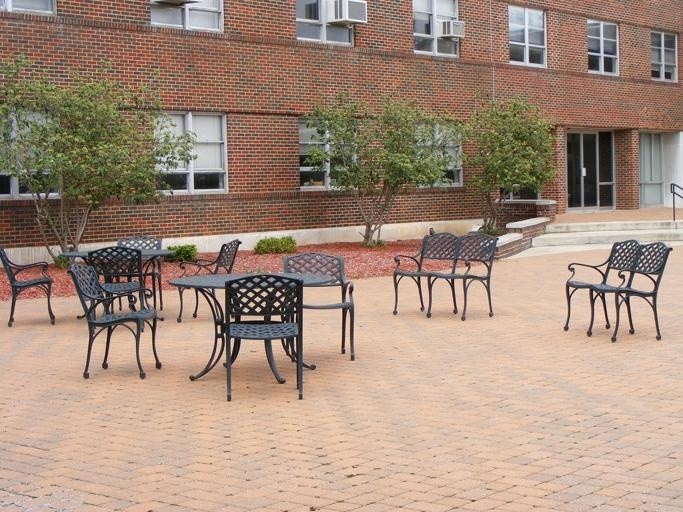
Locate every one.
[563,240,672,343]
[392,232,499,321]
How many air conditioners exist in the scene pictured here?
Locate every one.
[330,0,367,28]
[439,21,465,39]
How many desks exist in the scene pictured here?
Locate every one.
[60,250,178,310]
[167,273,334,385]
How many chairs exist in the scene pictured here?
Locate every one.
[68,262,162,380]
[176,239,242,323]
[77,236,163,333]
[0,246,56,327]
[283,252,355,361]
[222,274,303,402]
